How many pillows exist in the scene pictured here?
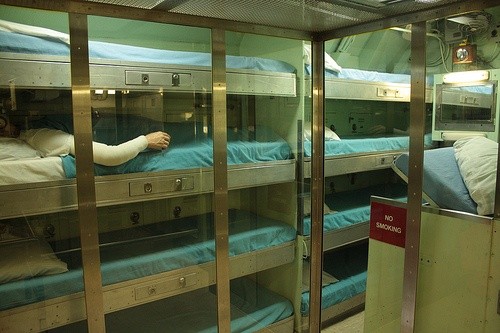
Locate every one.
[0,240,68,285]
[304,192,336,218]
[304,43,341,73]
[0,138,43,161]
[301,261,339,294]
[304,120,341,142]
[452,135,499,216]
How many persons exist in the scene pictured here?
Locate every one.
[0,107,171,165]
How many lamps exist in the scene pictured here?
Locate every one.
[108,90,116,94]
[94,89,104,96]
[443,71,491,84]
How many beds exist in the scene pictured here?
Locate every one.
[0,0,500,333]
